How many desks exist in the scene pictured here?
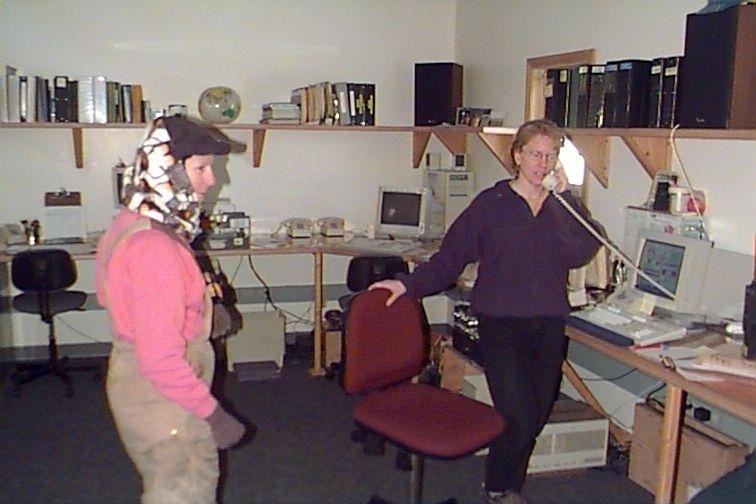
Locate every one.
[415,251,756,504]
[0,234,439,379]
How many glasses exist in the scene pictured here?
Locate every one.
[523,149,558,164]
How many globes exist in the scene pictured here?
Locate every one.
[198,86,242,124]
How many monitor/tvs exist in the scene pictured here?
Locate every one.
[376,186,445,242]
[623,228,755,322]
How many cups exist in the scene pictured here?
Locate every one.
[669,185,691,215]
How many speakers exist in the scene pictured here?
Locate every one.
[414,63,463,125]
[427,153,441,170]
[674,4,756,129]
[452,154,467,171]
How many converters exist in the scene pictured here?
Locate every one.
[693,408,711,422]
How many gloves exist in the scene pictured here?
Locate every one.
[204,403,247,449]
[211,303,232,339]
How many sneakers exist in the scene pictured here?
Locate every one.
[481,482,528,504]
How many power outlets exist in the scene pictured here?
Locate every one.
[682,401,722,430]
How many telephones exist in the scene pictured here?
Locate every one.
[317,216,345,237]
[542,158,563,191]
[284,217,313,238]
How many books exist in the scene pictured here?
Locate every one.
[258,80,377,127]
[541,55,686,128]
[0,65,148,124]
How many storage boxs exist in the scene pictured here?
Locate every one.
[630,403,744,500]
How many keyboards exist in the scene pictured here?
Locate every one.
[343,236,414,254]
[572,301,688,346]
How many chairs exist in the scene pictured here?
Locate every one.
[10,250,98,403]
[341,289,503,502]
[318,254,406,394]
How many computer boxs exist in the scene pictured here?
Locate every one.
[527,400,610,476]
[424,171,474,237]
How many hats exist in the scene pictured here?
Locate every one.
[163,113,231,158]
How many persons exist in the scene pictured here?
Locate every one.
[91,114,250,504]
[367,115,604,504]
